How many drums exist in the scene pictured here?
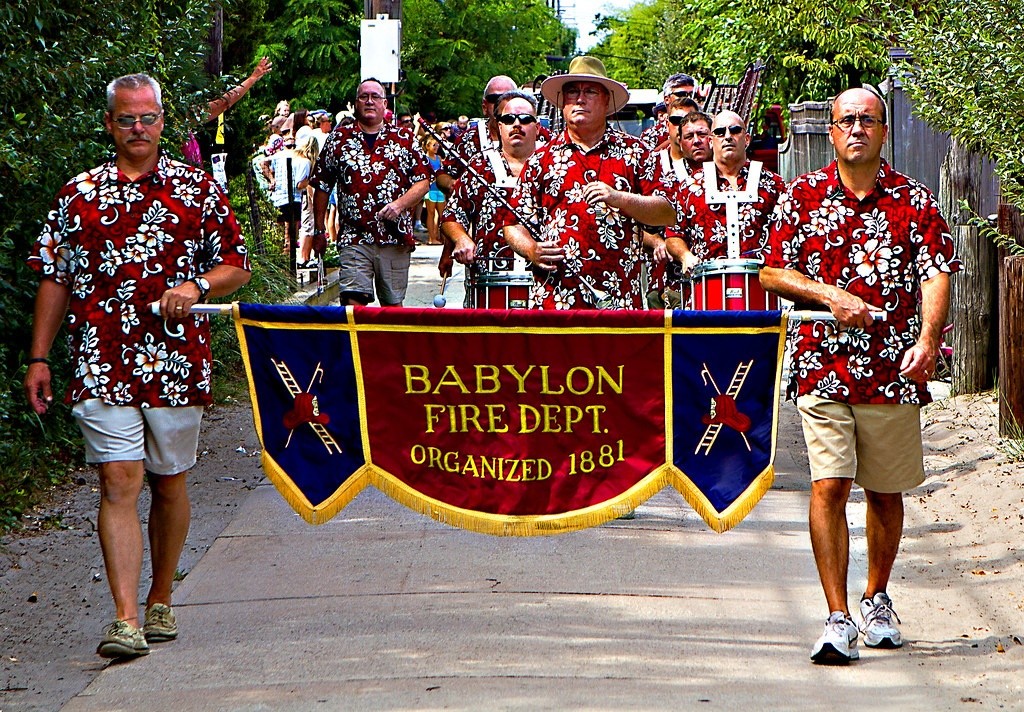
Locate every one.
[687,259,784,315]
[468,269,534,310]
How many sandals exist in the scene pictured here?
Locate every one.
[297,259,318,269]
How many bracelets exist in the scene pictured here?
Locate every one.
[24,357,51,366]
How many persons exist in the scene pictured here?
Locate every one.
[181,55,272,169]
[759,86,965,661]
[255,74,785,310]
[499,55,677,518]
[24,74,254,658]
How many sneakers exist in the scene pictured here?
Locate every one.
[810,611,860,662]
[858,592,902,649]
[144,603,179,641]
[414,224,428,232]
[97,618,150,657]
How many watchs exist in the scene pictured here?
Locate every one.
[187,276,211,302]
[313,228,326,235]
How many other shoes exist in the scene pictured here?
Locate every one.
[413,240,422,245]
[428,240,442,244]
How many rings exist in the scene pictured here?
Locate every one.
[597,190,601,196]
[175,306,183,310]
[388,215,392,219]
[263,67,266,70]
[924,368,928,375]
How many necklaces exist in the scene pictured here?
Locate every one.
[570,144,604,207]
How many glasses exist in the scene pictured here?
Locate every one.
[565,86,606,101]
[485,94,502,104]
[356,93,385,102]
[668,116,683,125]
[669,90,694,99]
[401,119,413,123]
[321,119,331,123]
[429,143,439,146]
[110,111,161,130]
[283,143,297,149]
[710,126,747,136]
[832,117,884,127]
[497,114,537,125]
[440,127,451,131]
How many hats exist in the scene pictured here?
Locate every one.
[542,56,629,116]
[317,109,326,114]
